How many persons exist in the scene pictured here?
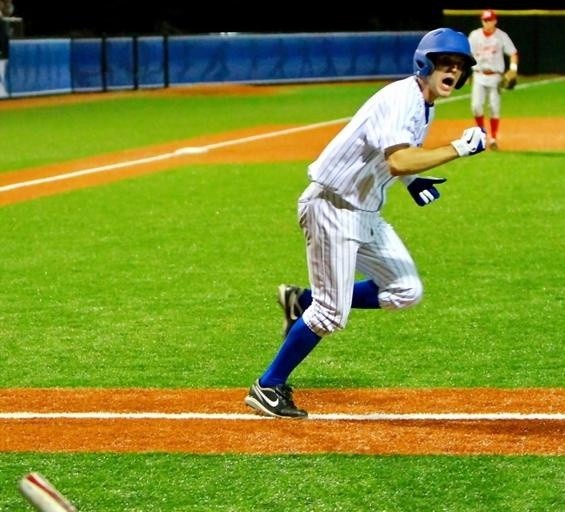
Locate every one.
[0,1,25,100]
[244,26,487,420]
[466,10,519,153]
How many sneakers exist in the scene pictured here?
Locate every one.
[277,283,304,338]
[490,141,498,150]
[244,378,309,419]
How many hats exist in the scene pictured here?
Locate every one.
[481,11,497,21]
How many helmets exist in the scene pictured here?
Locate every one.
[412,28,477,89]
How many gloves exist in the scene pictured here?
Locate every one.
[451,125,487,158]
[399,174,447,208]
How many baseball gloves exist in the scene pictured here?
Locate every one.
[499,69,517,89]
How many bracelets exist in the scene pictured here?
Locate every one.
[508,64,518,72]
